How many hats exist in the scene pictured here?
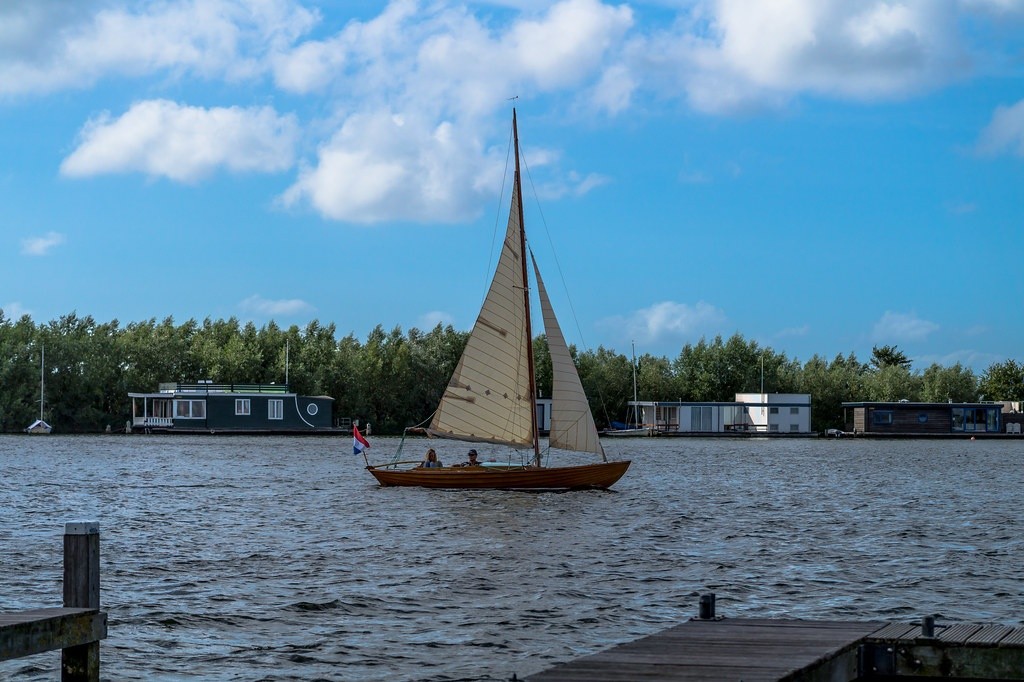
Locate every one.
[468,449,478,455]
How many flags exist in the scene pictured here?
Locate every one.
[353,425,370,456]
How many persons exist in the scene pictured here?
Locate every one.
[423,449,443,468]
[461,449,483,467]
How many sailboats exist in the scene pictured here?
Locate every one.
[363,96,633,491]
[606,339,650,437]
[27,339,52,435]
